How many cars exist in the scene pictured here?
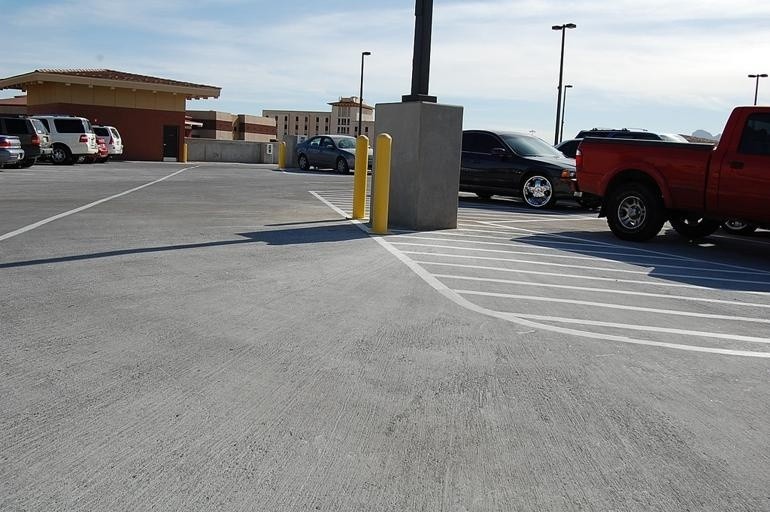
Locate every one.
[457,129,692,212]
[294,133,375,174]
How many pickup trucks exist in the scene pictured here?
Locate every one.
[574,106,770,240]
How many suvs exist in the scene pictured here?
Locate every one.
[0,113,125,169]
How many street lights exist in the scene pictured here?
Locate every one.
[550,21,576,146]
[559,84,573,144]
[748,73,768,105]
[357,50,372,136]
